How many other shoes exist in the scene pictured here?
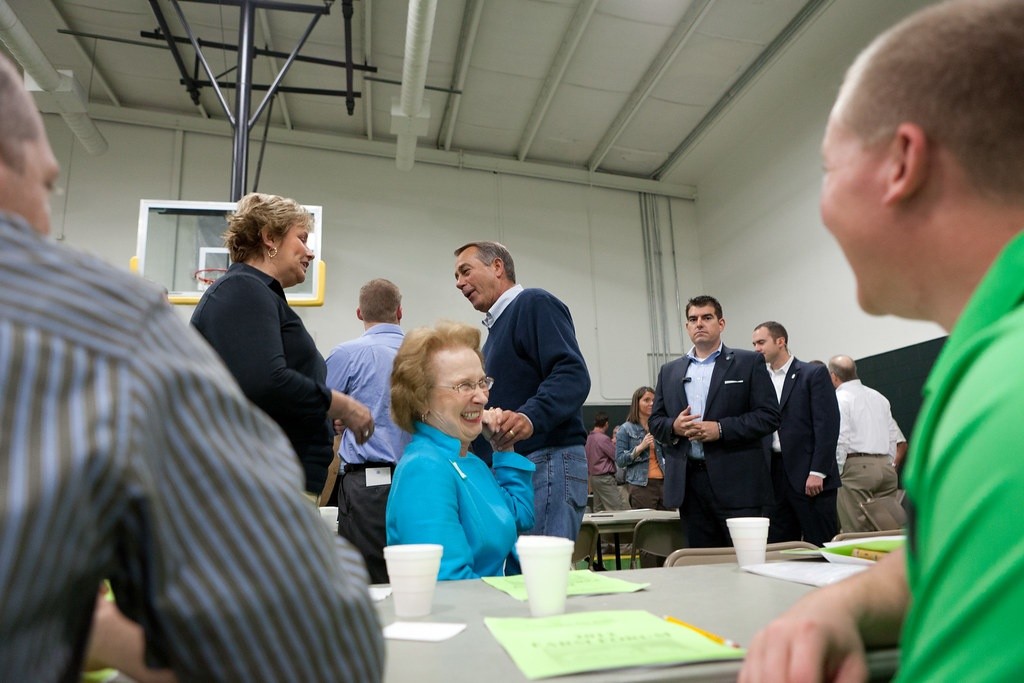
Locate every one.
[620,547,639,554]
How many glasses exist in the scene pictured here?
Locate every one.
[429,377,494,396]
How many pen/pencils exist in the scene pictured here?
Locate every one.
[663,615,739,649]
[591,515,613,517]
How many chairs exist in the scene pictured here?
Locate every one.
[571,494,909,567]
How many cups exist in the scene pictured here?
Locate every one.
[384,544,443,618]
[516,535,575,616]
[318,506,339,529]
[726,517,770,564]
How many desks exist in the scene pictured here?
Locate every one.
[581,511,680,570]
[368,560,899,683]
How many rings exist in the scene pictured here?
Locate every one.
[509,430,515,436]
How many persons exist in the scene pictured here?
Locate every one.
[586,411,626,511]
[735,1,1024,683]
[0,51,390,681]
[612,426,633,510]
[828,354,909,533]
[383,314,539,582]
[614,384,676,511]
[646,295,784,546]
[186,189,373,505]
[452,241,592,577]
[324,277,417,584]
[751,320,845,549]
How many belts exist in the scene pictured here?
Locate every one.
[344,461,395,473]
[687,460,706,471]
[592,472,615,476]
[847,452,889,457]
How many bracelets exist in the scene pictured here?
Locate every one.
[716,420,722,439]
